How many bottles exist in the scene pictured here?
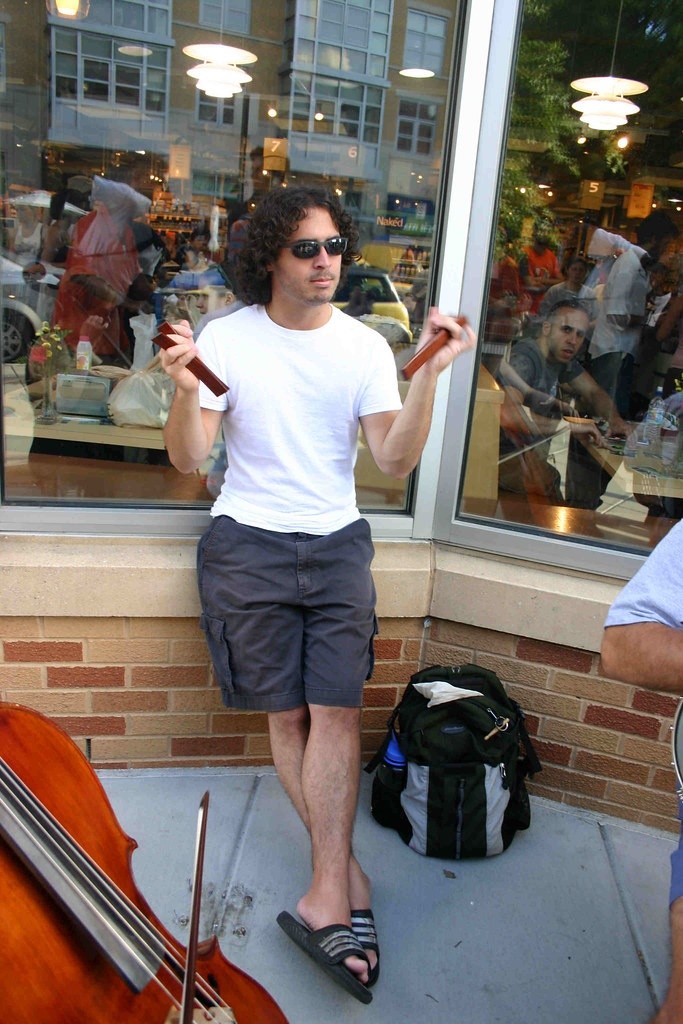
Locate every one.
[77,336,94,370]
[645,387,666,454]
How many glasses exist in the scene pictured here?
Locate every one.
[280,237,350,260]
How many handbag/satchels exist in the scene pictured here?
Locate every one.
[90,352,176,429]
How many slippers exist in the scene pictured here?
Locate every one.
[275,908,381,1005]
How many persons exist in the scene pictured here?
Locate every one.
[0,145,293,500]
[486,207,683,518]
[601,509,683,1024]
[165,186,474,989]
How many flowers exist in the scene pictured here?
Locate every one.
[29,323,73,364]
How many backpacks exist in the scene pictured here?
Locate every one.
[363,663,543,858]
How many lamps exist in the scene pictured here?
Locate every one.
[170,143,192,182]
[570,0,649,132]
[180,1,255,98]
[46,0,90,20]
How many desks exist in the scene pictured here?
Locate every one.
[581,418,682,501]
[2,366,179,450]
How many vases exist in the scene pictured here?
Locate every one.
[34,358,61,424]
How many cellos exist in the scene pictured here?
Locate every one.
[0,703,288,1023]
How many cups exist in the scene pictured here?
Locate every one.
[661,436,681,467]
